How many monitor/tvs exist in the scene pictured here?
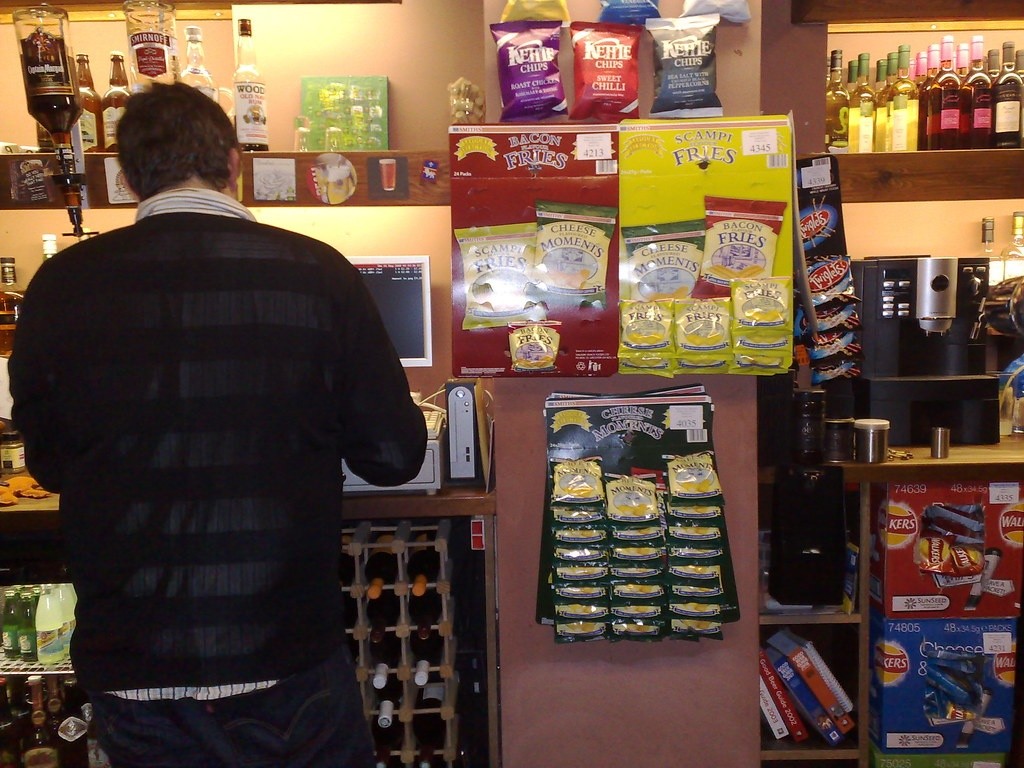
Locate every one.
[343,256,433,367]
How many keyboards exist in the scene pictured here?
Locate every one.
[421,411,444,439]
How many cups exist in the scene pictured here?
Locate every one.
[792,388,951,465]
[379,159,396,191]
[987,370,1024,438]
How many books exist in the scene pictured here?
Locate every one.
[759,628,855,746]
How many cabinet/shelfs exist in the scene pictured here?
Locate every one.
[757,424,1024,768]
[789,0,1024,203]
[342,519,460,768]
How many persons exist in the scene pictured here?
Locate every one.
[7,83,428,768]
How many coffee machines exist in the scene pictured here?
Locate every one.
[850,252,1001,445]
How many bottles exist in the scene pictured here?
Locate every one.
[338,518,488,768]
[1,234,59,358]
[12,0,234,241]
[0,584,111,768]
[980,210,1023,287]
[234,18,269,151]
[825,34,1024,150]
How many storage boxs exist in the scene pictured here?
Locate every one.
[868,609,1017,753]
[869,741,1005,768]
[869,478,1024,618]
